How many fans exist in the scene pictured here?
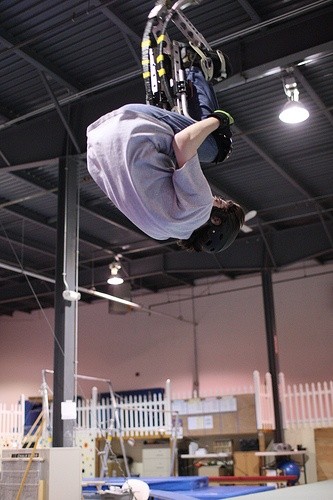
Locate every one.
[241,209,259,235]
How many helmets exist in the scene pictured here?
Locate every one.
[182,201,246,255]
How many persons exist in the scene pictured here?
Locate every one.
[86,49,245,256]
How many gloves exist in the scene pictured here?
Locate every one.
[207,108,235,127]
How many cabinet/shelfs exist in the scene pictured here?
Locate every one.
[142,445,174,478]
[234,451,261,484]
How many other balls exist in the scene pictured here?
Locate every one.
[276,459,300,485]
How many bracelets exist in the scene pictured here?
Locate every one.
[206,112,230,132]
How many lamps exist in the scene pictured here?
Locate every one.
[127,438,135,447]
[107,252,125,285]
[276,75,310,124]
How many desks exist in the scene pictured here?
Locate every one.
[255,451,308,484]
[180,453,227,458]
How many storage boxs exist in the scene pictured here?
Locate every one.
[199,465,226,488]
[1,448,82,499]
[99,458,126,477]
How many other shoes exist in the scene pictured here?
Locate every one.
[189,51,231,81]
